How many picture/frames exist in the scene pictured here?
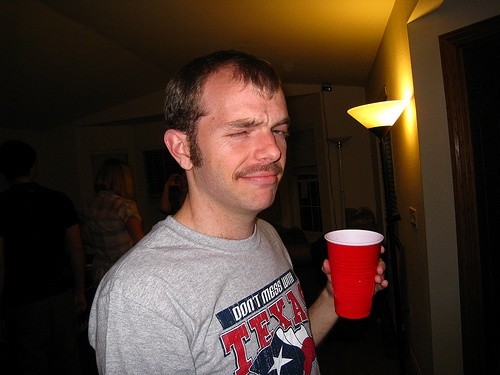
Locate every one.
[89,150,128,193]
[140,147,186,202]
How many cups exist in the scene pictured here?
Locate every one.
[324,229,385,319]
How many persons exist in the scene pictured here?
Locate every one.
[87,157,145,298]
[0,140,87,375]
[87,48,389,375]
[160,159,189,215]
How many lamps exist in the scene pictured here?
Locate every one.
[347,99,410,375]
